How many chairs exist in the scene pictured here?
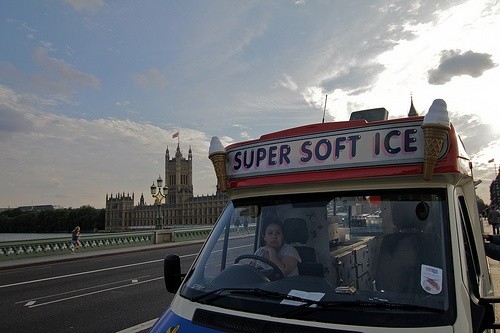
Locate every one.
[375,202,440,292]
[283,218,325,276]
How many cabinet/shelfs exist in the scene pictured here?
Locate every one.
[335,244,369,292]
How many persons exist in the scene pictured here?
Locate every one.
[69,226,82,255]
[488,205,500,234]
[250,219,302,280]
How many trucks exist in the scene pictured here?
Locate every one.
[149,96,499,332]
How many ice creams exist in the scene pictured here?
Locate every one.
[421,99,451,181]
